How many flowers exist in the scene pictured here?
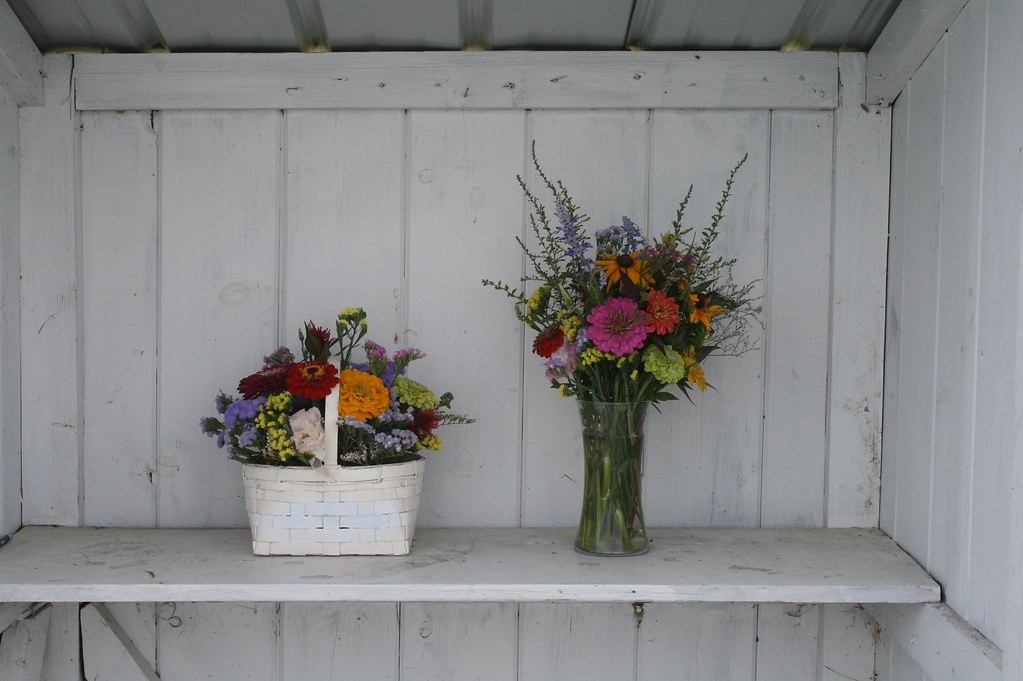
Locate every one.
[200,304,476,466]
[480,139,766,550]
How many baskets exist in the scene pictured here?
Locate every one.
[242,354,426,556]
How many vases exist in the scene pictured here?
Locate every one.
[572,396,654,558]
